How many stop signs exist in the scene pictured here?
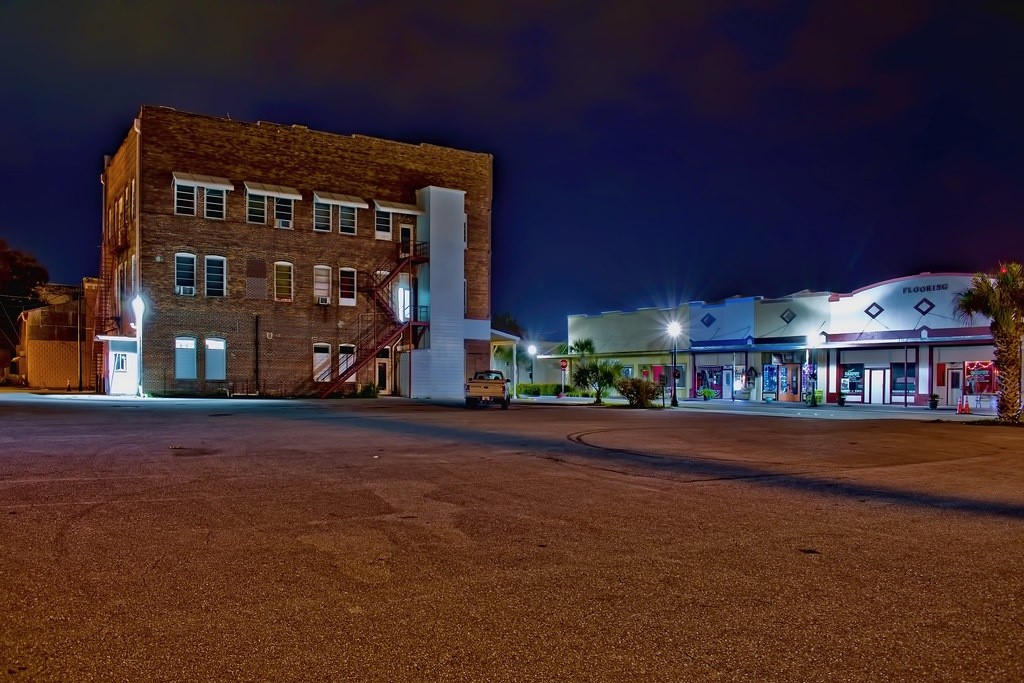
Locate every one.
[560,359,567,368]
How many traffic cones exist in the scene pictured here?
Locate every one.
[955,395,964,414]
[964,395,972,415]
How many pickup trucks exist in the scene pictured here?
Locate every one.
[464,369,511,410]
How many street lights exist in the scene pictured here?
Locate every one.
[669,322,679,407]
[528,344,537,384]
[808,330,819,407]
